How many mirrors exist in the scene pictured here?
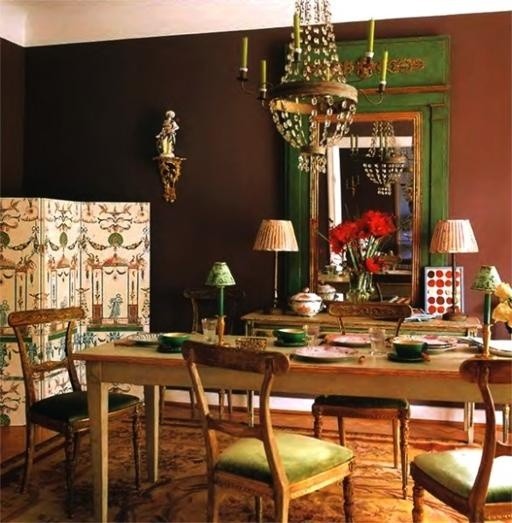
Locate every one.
[307,108,425,313]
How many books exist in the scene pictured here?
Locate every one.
[456,335,512,356]
[424,266,464,315]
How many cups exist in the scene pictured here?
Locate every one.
[369,327,385,357]
[201,317,218,341]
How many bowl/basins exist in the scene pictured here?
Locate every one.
[389,336,424,357]
[317,280,336,300]
[159,331,192,347]
[274,329,304,342]
[288,287,323,317]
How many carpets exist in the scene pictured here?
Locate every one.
[2,414,483,521]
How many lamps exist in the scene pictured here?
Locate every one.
[345,116,411,195]
[426,217,481,321]
[236,1,392,173]
[253,217,300,314]
[469,263,501,359]
[204,259,237,349]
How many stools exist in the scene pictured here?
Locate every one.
[459,396,511,444]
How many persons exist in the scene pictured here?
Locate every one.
[109,295,123,319]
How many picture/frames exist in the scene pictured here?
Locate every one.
[419,262,466,320]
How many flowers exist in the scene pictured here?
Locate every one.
[490,279,512,334]
[322,206,393,275]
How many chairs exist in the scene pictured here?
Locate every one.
[309,297,415,498]
[3,301,147,520]
[182,283,248,423]
[408,350,512,522]
[180,336,356,522]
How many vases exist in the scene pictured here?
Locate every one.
[343,264,374,301]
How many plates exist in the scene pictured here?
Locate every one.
[274,340,306,346]
[324,332,371,347]
[292,345,358,360]
[478,343,512,357]
[387,351,425,361]
[414,335,457,354]
[157,345,182,353]
[127,333,159,345]
